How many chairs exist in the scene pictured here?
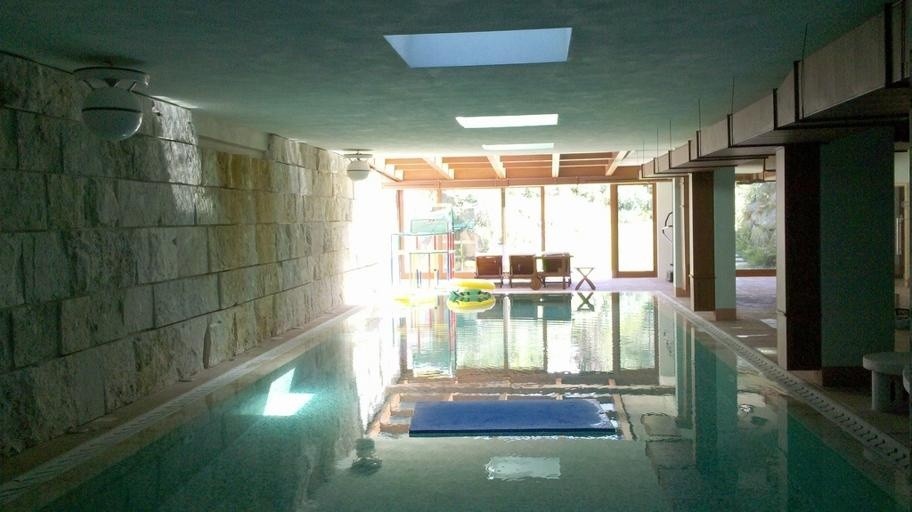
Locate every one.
[472,252,574,290]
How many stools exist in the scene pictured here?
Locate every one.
[862,351,910,412]
[574,267,597,290]
[575,292,595,311]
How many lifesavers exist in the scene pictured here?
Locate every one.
[446,295,496,313]
[450,278,495,292]
[391,295,438,311]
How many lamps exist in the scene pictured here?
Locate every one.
[72,67,152,142]
[342,151,373,181]
